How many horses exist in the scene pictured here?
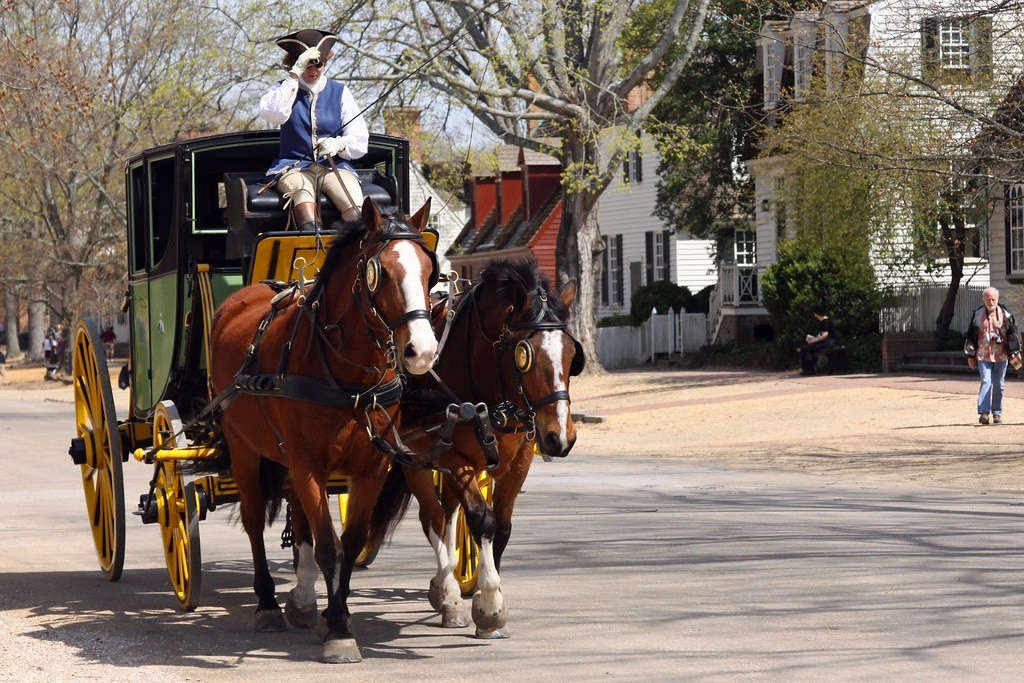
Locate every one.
[364,252,577,638]
[210,195,439,664]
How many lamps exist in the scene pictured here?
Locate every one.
[761,200,770,212]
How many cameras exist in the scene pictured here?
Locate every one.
[992,336,1002,344]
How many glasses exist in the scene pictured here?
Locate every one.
[315,62,324,68]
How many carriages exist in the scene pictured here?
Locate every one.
[64,130,586,665]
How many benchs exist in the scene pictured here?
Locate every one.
[796,346,852,375]
[223,166,400,258]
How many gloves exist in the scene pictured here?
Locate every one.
[315,136,345,159]
[289,47,321,77]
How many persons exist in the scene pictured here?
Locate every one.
[260,29,370,229]
[964,287,1021,424]
[43,323,63,380]
[800,307,833,375]
[100,326,116,362]
[0,352,9,384]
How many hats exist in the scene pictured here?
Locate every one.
[276,29,339,68]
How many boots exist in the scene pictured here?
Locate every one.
[295,202,323,231]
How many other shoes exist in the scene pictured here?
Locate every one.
[45,373,57,381]
[979,414,989,424]
[800,370,816,376]
[993,415,1002,423]
[3,380,11,385]
